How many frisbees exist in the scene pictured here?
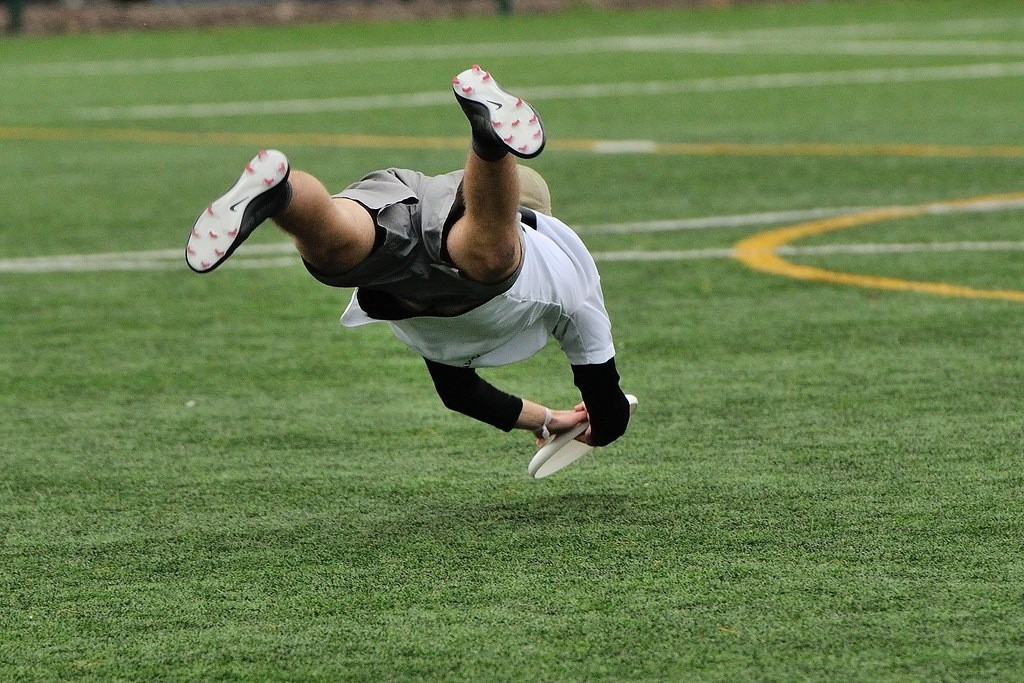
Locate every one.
[526,393,640,481]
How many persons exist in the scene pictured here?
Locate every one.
[184,64,631,448]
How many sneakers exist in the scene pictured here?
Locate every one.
[451,64,546,159]
[185,148,290,275]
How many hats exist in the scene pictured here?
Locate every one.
[515,164,552,217]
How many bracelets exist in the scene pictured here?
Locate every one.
[533,406,553,440]
